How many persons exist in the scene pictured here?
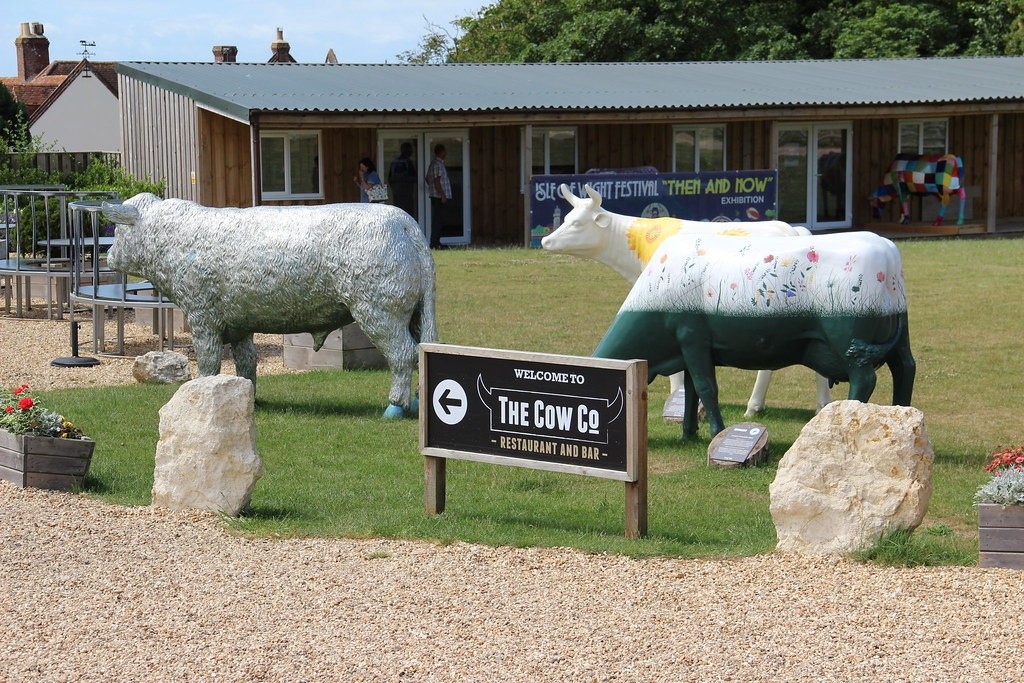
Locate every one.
[388,143,416,215]
[353,158,382,203]
[425,144,452,249]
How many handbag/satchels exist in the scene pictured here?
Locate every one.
[364,176,389,203]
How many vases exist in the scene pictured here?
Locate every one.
[0,429,95,489]
[978,504,1024,569]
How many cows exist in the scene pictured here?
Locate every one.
[539,183,834,422]
[867,152,967,227]
[100,192,438,422]
[590,227,919,445]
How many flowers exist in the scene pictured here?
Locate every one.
[975,446,1024,506]
[0,384,83,439]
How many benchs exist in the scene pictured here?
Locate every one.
[0,238,179,353]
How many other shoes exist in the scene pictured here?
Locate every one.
[430,243,447,250]
